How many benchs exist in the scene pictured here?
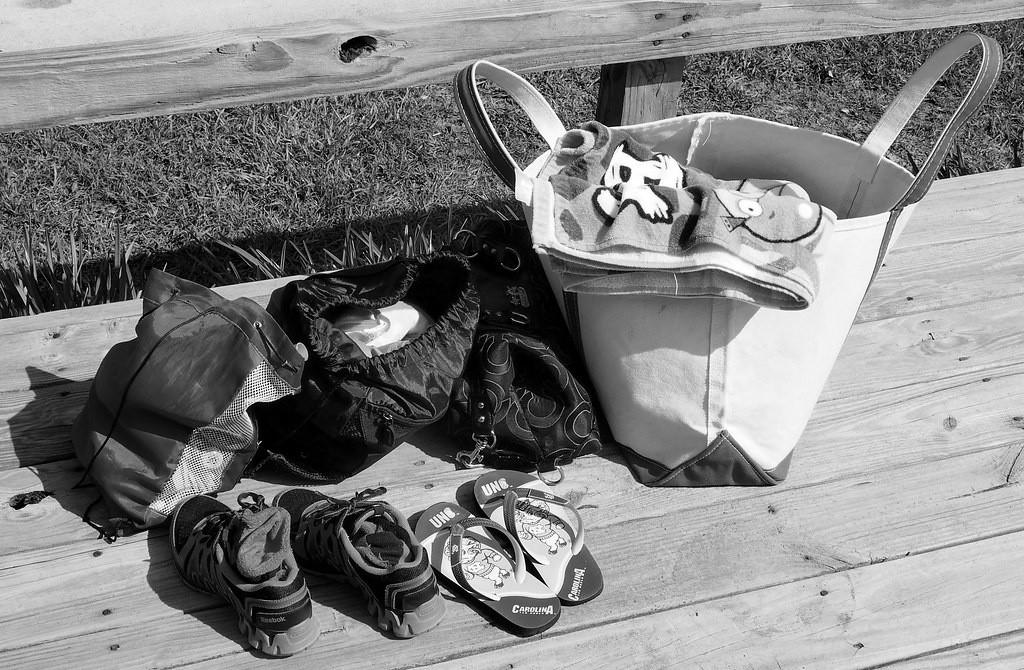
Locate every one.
[0,0,1024,670]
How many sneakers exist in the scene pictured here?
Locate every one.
[273,487,448,639]
[169,493,323,657]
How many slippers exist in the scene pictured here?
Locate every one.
[474,470,604,606]
[415,502,561,637]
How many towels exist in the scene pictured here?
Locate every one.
[526,118,839,312]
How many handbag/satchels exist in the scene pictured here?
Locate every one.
[448,205,603,473]
[453,33,1004,487]
